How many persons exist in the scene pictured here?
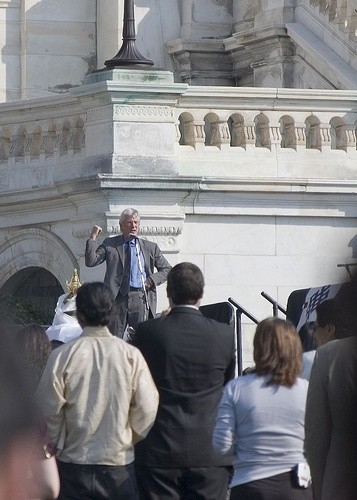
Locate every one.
[299,277,357,500]
[213,317,315,500]
[132,263,236,500]
[34,283,160,500]
[0,321,61,500]
[85,208,173,339]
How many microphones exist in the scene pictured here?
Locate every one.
[129,233,138,239]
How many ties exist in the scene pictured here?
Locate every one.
[120,242,132,297]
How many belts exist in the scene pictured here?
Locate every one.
[130,288,140,291]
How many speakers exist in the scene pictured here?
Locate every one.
[200,302,235,330]
